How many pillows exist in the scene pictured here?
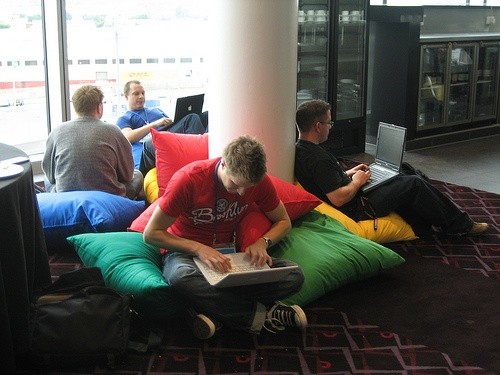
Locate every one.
[149,127,209,198]
[36,190,146,251]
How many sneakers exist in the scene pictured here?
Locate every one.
[183,306,216,340]
[262,300,308,334]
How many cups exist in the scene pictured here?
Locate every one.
[298,9,327,23]
[342,10,360,24]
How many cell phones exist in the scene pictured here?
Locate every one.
[214,248,236,254]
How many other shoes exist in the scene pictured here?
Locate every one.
[470,222,488,233]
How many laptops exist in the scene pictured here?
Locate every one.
[193,251,299,288]
[157,93,205,131]
[360,122,407,194]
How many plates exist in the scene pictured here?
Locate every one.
[0,164,23,179]
[0,156,29,164]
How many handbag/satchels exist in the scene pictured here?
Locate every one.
[28,267,130,369]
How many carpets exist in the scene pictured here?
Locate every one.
[47,158,500,375]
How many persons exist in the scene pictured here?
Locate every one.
[294,100,488,237]
[143,136,308,340]
[41,85,143,200]
[116,80,173,169]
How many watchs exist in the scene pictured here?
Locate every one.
[262,236,272,249]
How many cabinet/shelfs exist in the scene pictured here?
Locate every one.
[413,33,500,149]
[298,0,370,156]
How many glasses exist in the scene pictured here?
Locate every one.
[98,100,106,104]
[312,120,334,129]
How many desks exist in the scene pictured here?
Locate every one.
[0,143,53,375]
